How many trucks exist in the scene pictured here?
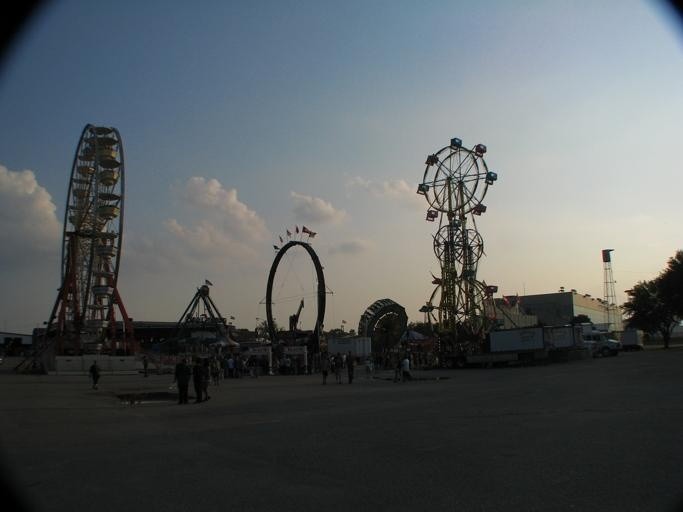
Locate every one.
[490,322,645,360]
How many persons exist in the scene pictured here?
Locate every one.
[173,353,254,405]
[143,355,149,377]
[89,360,102,390]
[273,355,292,376]
[318,351,354,384]
[392,353,411,384]
